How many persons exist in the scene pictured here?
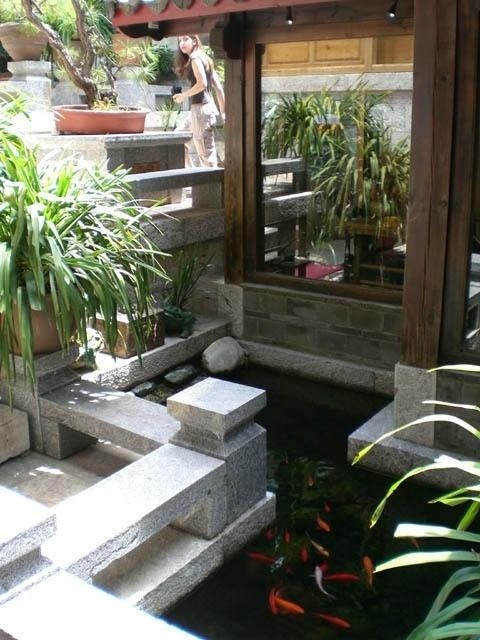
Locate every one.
[173,34,225,198]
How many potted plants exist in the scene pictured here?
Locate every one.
[0,0,52,60]
[15,0,166,135]
[154,241,218,338]
[0,85,181,416]
[307,89,411,263]
